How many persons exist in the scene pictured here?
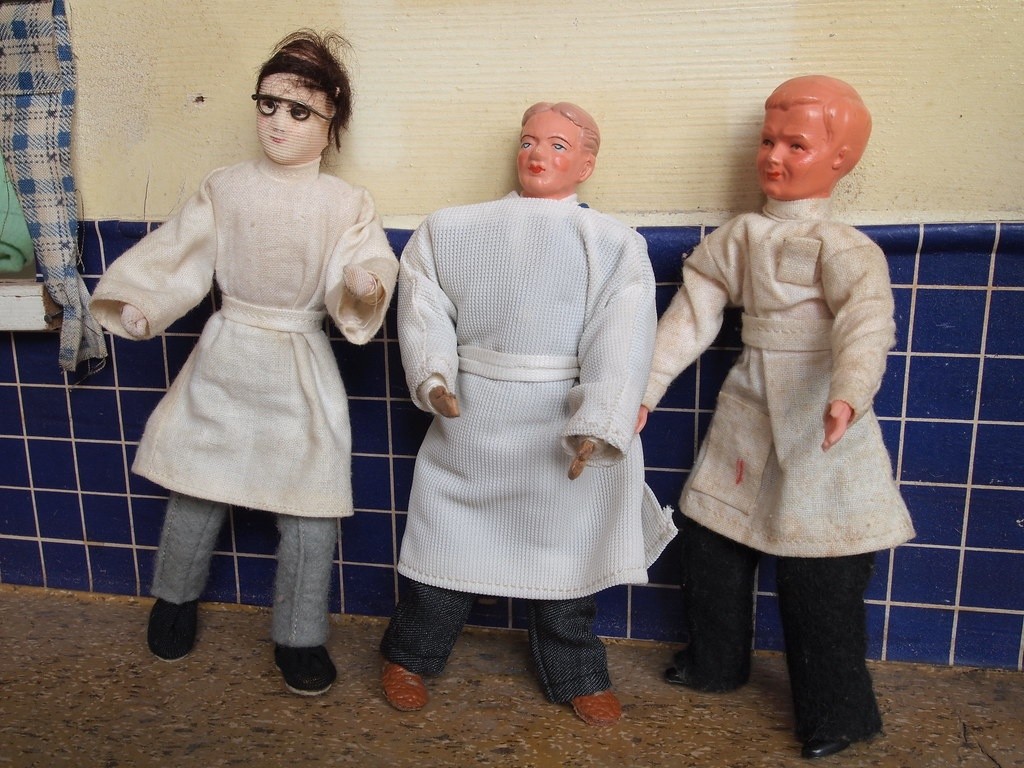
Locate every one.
[379,102,678,727]
[88,25,399,697]
[634,75,919,760]
